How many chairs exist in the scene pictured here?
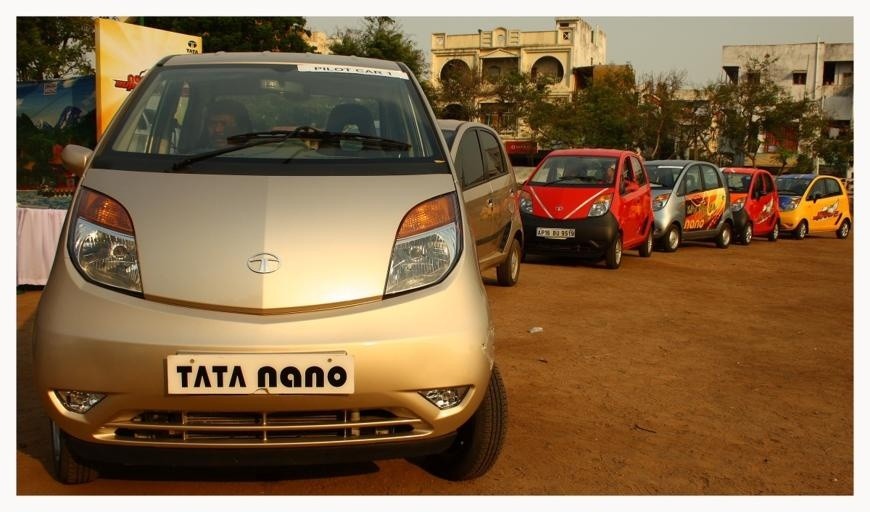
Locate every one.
[318,103,383,151]
[683,175,695,194]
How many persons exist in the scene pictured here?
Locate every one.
[192,99,254,155]
[597,164,639,194]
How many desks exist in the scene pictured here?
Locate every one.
[16,206,68,287]
